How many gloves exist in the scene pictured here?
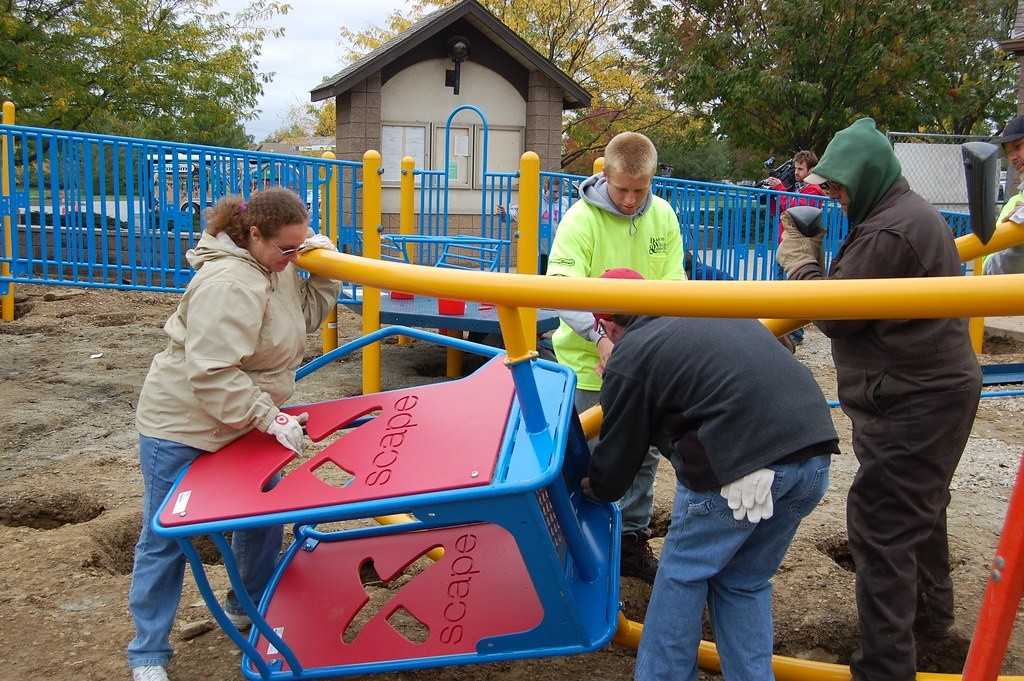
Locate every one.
[267,412,309,458]
[305,234,338,252]
[733,488,774,523]
[721,468,775,510]
[776,212,827,280]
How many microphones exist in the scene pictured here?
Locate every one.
[753,181,763,188]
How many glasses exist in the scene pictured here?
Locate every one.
[597,325,608,338]
[270,239,309,256]
[826,182,842,189]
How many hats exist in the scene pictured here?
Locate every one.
[803,174,827,185]
[991,114,1024,144]
[593,269,643,332]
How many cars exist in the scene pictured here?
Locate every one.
[149,162,231,216]
[238,163,299,198]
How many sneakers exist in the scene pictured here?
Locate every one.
[619,531,658,586]
[133,665,172,681]
[223,610,252,630]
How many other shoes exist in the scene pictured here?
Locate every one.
[793,338,803,346]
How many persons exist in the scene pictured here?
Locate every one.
[982,114,1024,275]
[547,130,688,586]
[778,117,982,681]
[128,187,341,681]
[581,269,842,681]
[767,151,828,345]
